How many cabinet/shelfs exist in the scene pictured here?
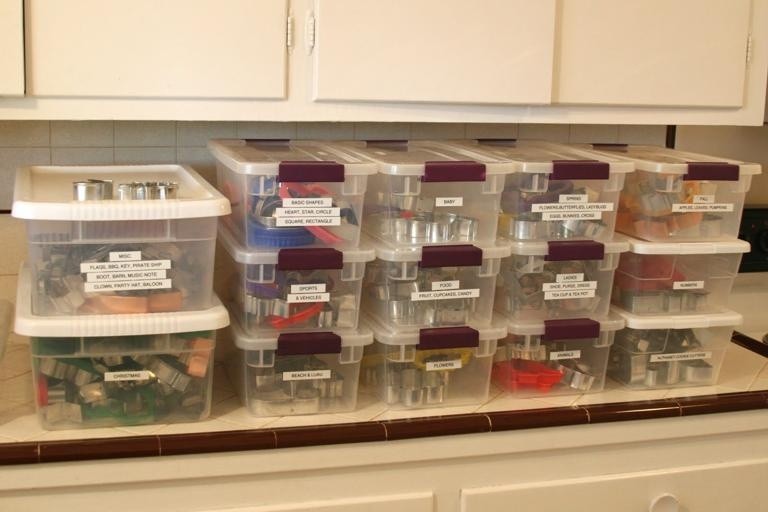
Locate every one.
[0,0,767,127]
[0,408,768,512]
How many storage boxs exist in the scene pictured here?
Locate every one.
[10,164,232,312]
[224,314,374,416]
[452,140,636,241]
[491,310,627,400]
[365,234,510,334]
[217,228,377,335]
[495,242,631,321]
[365,318,507,406]
[564,143,761,240]
[14,262,231,429]
[208,140,379,250]
[605,306,744,390]
[608,230,755,314]
[322,137,515,247]
